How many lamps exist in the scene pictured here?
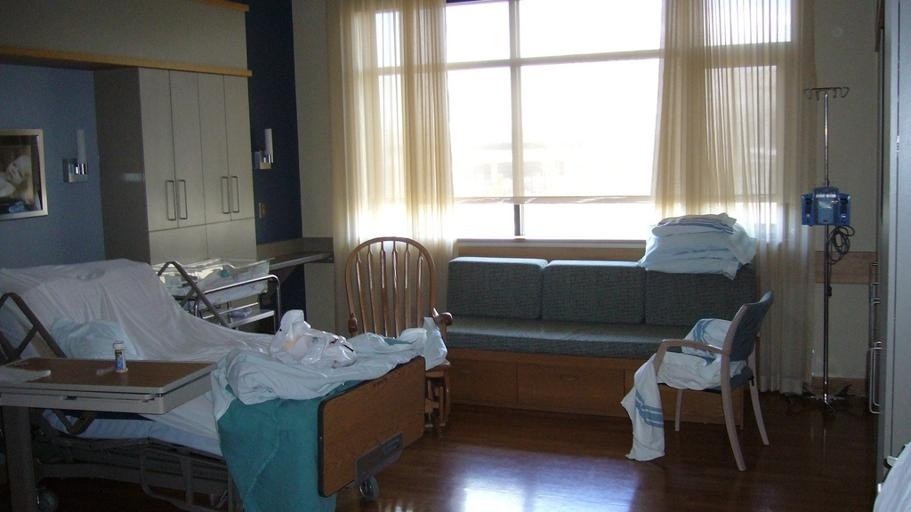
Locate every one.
[255,129,274,170]
[64,128,89,183]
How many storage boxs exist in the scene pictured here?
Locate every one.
[151,256,276,311]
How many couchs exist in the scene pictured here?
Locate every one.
[440,239,758,425]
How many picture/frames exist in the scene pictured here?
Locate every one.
[0,130,48,221]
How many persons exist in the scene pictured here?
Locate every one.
[1,154,35,206]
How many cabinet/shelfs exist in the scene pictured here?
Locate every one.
[876,1,911,482]
[93,68,261,333]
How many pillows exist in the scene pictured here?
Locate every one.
[645,262,756,327]
[448,258,547,319]
[544,259,647,325]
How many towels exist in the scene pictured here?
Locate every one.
[620,317,744,460]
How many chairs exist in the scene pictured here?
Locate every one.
[345,237,450,429]
[620,290,774,471]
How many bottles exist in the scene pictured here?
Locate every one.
[113,340,128,373]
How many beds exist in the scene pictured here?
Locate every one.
[0,258,425,512]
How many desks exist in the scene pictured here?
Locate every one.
[0,356,218,512]
